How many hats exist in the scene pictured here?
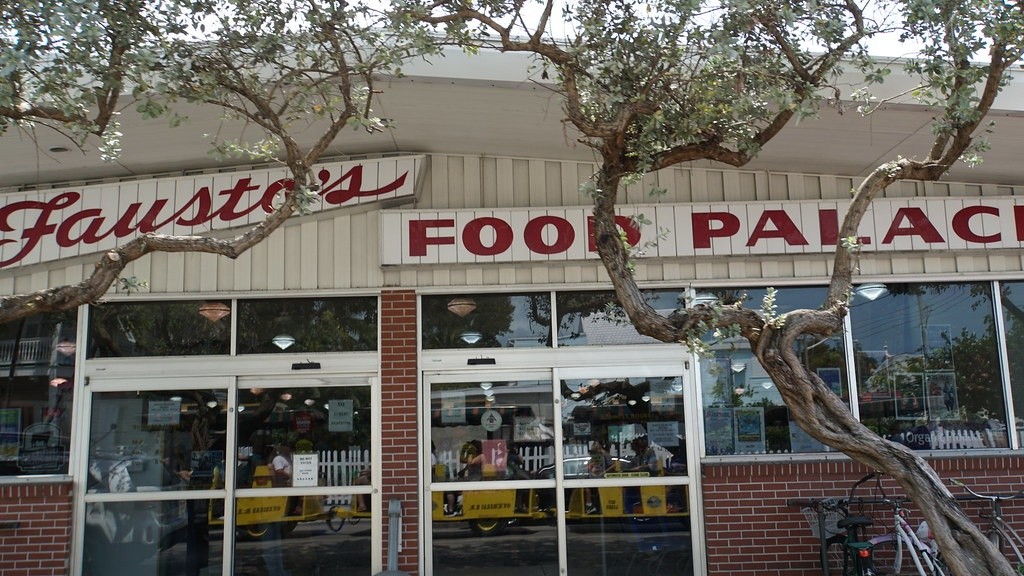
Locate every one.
[466,440,483,451]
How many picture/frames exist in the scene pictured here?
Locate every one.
[918,323,955,371]
[816,367,843,398]
[733,406,766,454]
[893,372,928,420]
[925,373,961,421]
[857,350,892,398]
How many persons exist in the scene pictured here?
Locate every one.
[444,439,484,514]
[237,442,293,488]
[355,465,371,512]
[507,433,688,515]
[431,440,437,465]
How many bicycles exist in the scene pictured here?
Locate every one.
[842,495,946,576]
[787,473,880,576]
[949,477,1024,564]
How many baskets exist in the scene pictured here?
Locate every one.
[802,496,850,539]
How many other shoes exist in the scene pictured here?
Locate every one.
[443,508,457,517]
[453,507,463,515]
[357,505,367,511]
[585,502,596,514]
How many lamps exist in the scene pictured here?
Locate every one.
[447,297,476,317]
[695,292,718,304]
[480,383,496,401]
[55,342,76,357]
[271,334,296,350]
[856,283,887,300]
[199,303,231,322]
[735,388,745,395]
[50,378,67,387]
[207,388,329,413]
[571,379,683,405]
[762,382,772,389]
[729,363,745,372]
[460,332,483,344]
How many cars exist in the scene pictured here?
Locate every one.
[82,455,188,576]
[535,457,631,512]
